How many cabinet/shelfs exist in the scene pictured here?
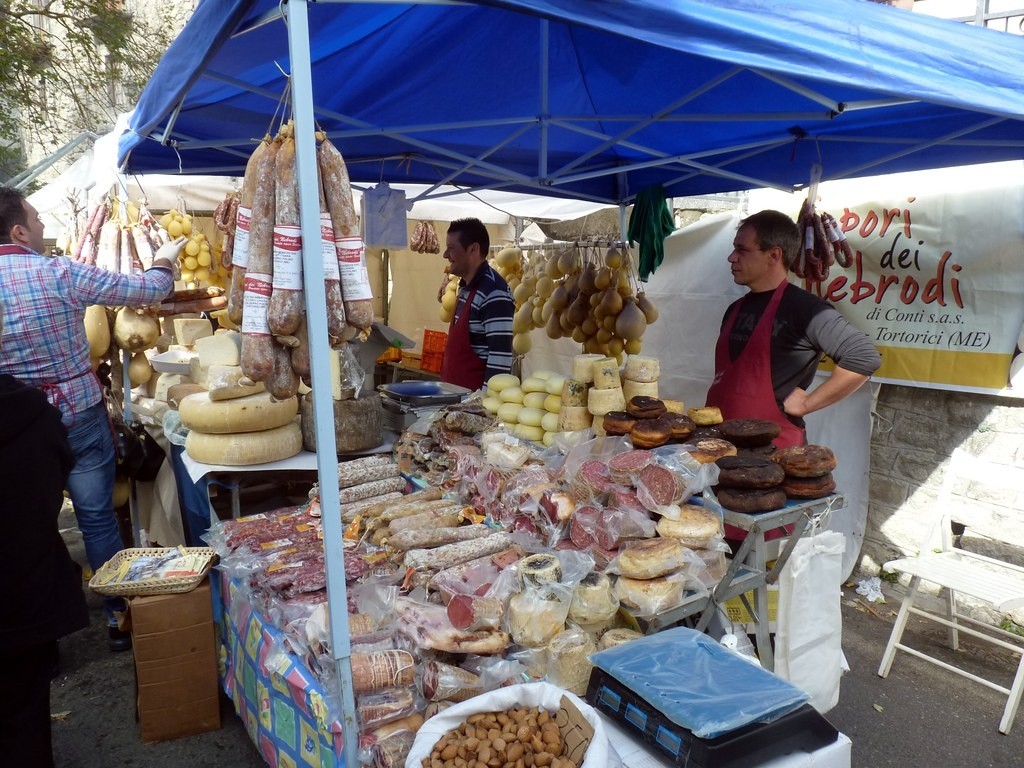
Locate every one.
[212,439,844,768]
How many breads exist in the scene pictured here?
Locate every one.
[614,505,722,614]
[482,353,838,514]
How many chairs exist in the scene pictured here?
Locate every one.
[878,447,1023,734]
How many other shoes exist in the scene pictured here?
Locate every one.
[107,624,131,651]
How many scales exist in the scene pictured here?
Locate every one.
[584,624,841,768]
[348,321,473,435]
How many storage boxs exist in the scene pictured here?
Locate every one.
[375,330,447,373]
[123,576,221,743]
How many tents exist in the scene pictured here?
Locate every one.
[24,0,1024,768]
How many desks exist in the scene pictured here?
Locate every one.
[387,361,440,383]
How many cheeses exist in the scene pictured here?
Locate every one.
[141,309,302,465]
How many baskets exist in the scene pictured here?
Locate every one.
[88,545,215,597]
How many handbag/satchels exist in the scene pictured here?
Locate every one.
[97,385,167,482]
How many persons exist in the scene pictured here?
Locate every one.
[704,211,882,571]
[442,218,515,391]
[0,188,189,653]
[0,375,91,768]
